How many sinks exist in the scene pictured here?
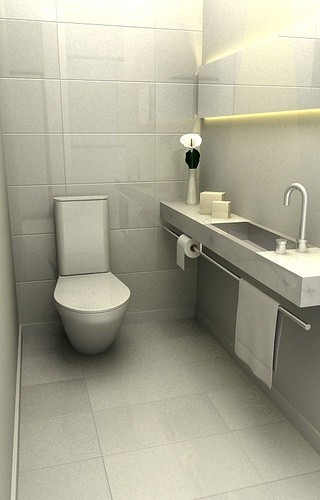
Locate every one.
[211,221,308,252]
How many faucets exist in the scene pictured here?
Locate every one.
[283,182,309,253]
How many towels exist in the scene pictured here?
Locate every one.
[233,278,283,391]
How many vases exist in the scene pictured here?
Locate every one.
[186,169,198,205]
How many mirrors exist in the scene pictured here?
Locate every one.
[198,22,320,119]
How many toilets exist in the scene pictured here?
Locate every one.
[52,195,132,356]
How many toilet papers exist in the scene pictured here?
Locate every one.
[176,233,200,271]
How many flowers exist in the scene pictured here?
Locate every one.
[179,133,202,169]
